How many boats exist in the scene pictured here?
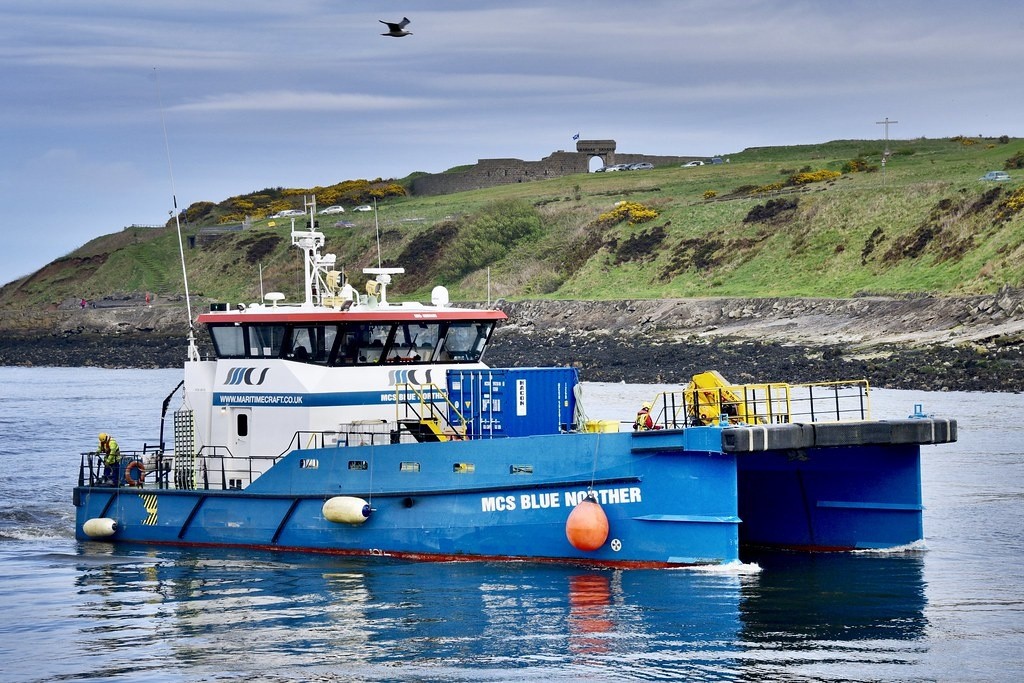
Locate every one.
[73,124,960,573]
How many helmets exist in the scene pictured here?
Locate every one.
[642,402,652,411]
[99,433,107,442]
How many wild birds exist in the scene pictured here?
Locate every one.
[379,17,413,37]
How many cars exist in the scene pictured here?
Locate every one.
[352,204,372,214]
[710,158,723,165]
[319,205,345,216]
[269,209,305,219]
[631,162,655,171]
[334,219,356,228]
[978,170,1011,182]
[595,163,636,175]
[680,161,706,167]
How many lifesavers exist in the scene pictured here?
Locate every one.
[124,460,146,486]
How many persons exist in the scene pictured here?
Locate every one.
[94,433,120,488]
[146,293,152,307]
[634,402,653,432]
[81,298,86,309]
